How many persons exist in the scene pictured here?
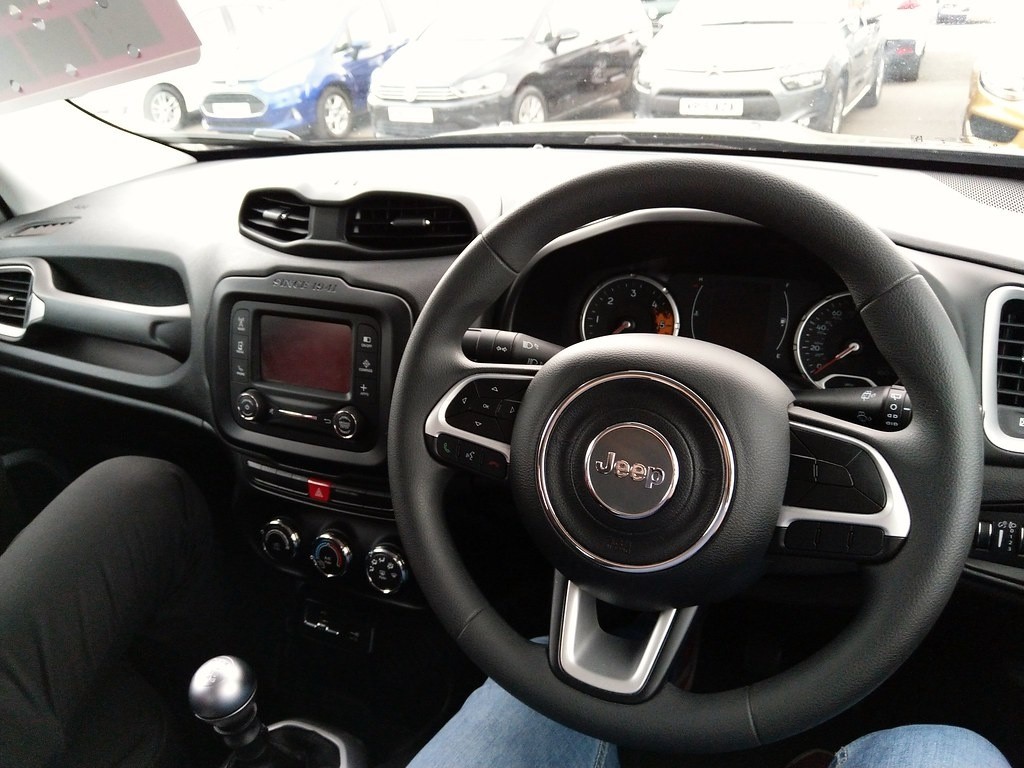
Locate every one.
[0,455,202,768]
[406,635,1013,768]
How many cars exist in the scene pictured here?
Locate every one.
[964,66,1024,148]
[68,39,274,133]
[936,2,971,24]
[634,1,888,142]
[885,8,927,82]
[194,29,411,142]
[366,0,655,129]
